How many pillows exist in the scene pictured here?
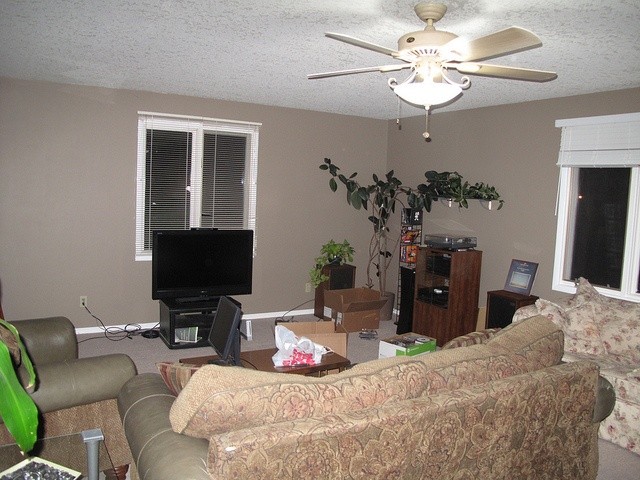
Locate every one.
[537,298,607,359]
[155,356,206,398]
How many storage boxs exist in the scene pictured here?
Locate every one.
[325,286,383,334]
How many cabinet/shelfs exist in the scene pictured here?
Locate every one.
[412,241,482,347]
[155,298,243,351]
[484,289,539,328]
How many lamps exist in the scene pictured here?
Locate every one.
[389,51,471,140]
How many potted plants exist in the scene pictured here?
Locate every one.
[318,155,505,321]
[307,239,354,288]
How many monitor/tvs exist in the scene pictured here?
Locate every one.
[150,228,253,301]
[203,296,244,363]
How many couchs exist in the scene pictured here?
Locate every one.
[512,278,639,455]
[116,314,618,479]
[1,309,137,479]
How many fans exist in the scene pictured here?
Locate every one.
[308,1,558,83]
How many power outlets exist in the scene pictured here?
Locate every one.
[79,296,87,310]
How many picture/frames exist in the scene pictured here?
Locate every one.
[504,258,538,295]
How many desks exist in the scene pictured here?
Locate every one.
[1,428,118,480]
